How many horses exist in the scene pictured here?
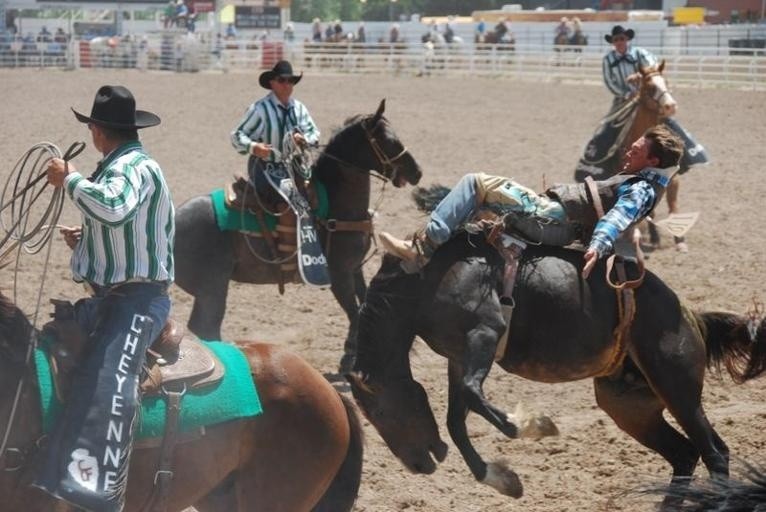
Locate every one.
[570,31,586,52]
[0,290,365,512]
[337,229,766,512]
[614,58,692,251]
[422,31,516,69]
[303,31,341,68]
[174,97,423,342]
[555,31,571,52]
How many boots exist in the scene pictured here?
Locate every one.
[378,230,440,275]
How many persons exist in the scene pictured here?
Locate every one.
[375,120,691,282]
[597,25,656,108]
[0,0,239,71]
[34,82,185,511]
[553,17,582,39]
[247,17,516,76]
[230,60,320,205]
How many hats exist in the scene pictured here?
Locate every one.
[605,26,634,43]
[70,85,161,128]
[260,61,302,89]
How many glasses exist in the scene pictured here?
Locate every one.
[274,76,295,84]
